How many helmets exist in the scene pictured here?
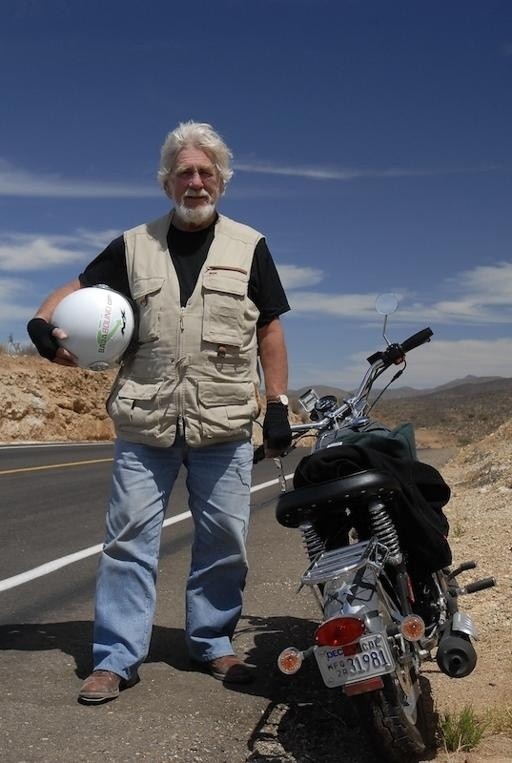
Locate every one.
[50,283,138,371]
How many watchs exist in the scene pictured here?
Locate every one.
[266,394,293,407]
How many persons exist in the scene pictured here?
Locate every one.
[24,121,299,707]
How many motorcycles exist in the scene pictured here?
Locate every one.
[251,289,497,759]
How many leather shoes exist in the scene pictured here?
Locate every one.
[79,669,140,703]
[191,654,253,683]
